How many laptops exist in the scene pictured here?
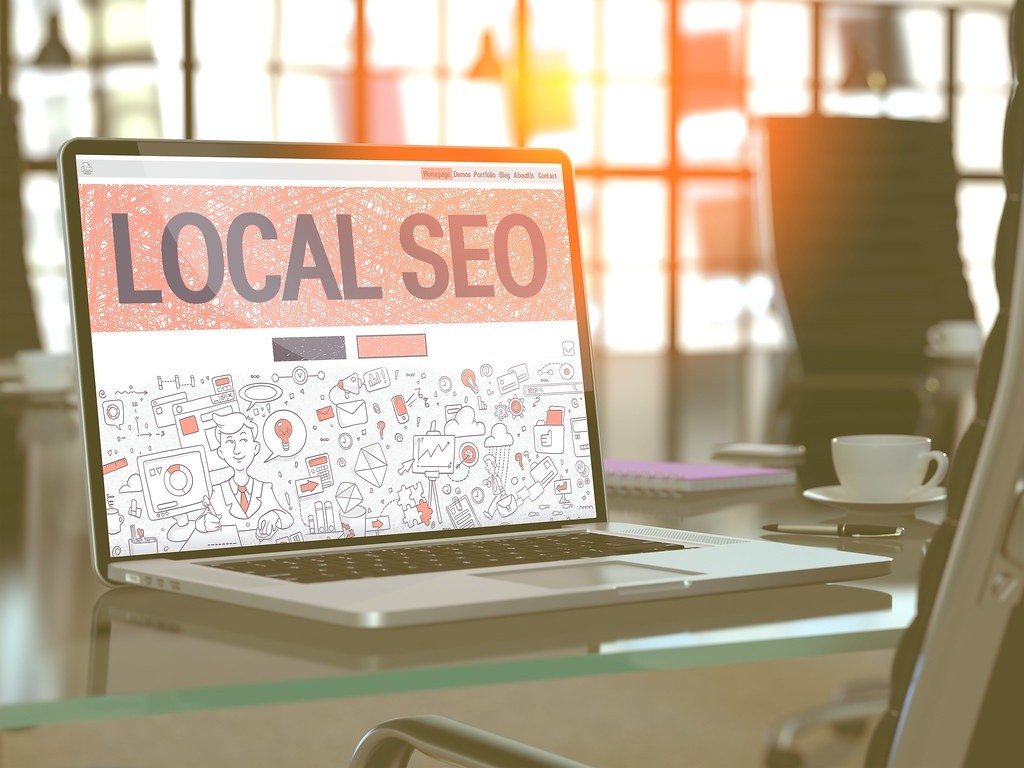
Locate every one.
[57,137,895,629]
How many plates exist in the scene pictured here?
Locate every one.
[802,482,950,516]
[711,440,805,465]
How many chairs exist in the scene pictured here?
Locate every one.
[343,0,1024,768]
[757,112,985,487]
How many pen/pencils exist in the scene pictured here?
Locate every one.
[761,522,905,540]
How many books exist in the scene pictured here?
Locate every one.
[713,442,807,468]
[602,460,799,500]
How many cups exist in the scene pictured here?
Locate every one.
[831,433,948,497]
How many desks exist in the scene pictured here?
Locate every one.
[0,352,977,768]
